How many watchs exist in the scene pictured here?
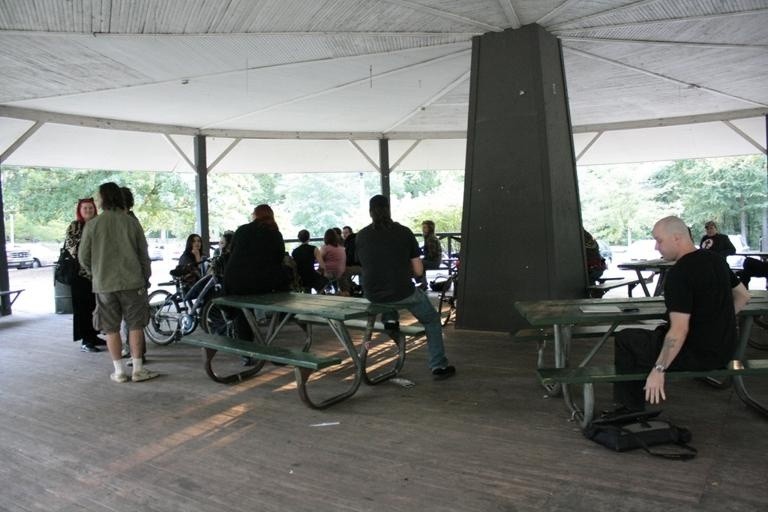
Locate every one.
[651,362,672,375]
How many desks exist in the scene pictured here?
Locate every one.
[513,287,767,390]
[203,290,409,410]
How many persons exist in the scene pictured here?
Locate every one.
[698,221,737,261]
[62,196,110,354]
[353,193,457,382]
[414,220,442,292]
[116,187,152,367]
[175,220,365,325]
[582,227,608,286]
[74,182,160,384]
[221,202,294,367]
[599,216,753,416]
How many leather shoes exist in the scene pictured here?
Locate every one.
[602,403,645,416]
[81,337,106,352]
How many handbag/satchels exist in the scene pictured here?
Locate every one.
[584,411,697,460]
[55,249,89,286]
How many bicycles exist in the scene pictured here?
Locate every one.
[436,251,461,329]
[139,252,256,347]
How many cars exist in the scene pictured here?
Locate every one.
[144,237,190,263]
[4,237,65,272]
[594,233,753,278]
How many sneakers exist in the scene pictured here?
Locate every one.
[433,365,455,380]
[110,349,160,383]
[385,321,398,331]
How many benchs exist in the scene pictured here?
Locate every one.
[575,238,768,294]
[511,322,707,367]
[292,314,428,383]
[536,356,768,436]
[179,330,350,410]
